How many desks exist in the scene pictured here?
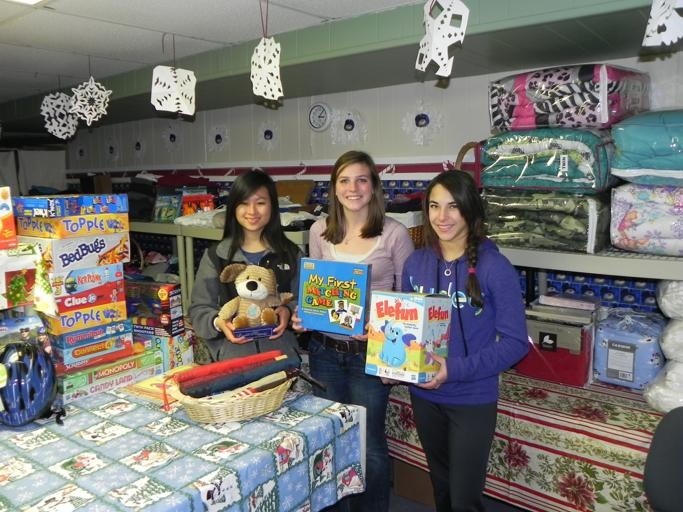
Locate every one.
[0,364,366,512]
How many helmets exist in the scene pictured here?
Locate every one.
[0,342,57,428]
[37,328,44,333]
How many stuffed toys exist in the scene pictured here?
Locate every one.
[216,262,294,331]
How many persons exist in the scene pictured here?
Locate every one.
[183,166,307,390]
[290,148,418,510]
[378,170,532,509]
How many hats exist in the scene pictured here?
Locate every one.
[19,328,28,332]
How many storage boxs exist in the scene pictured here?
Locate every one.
[509,292,663,388]
[364,287,451,385]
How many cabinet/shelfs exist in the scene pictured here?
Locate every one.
[129,221,311,326]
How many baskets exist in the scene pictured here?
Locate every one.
[168,378,296,423]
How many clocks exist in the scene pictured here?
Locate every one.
[307,104,331,132]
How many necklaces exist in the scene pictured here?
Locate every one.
[338,222,375,250]
[441,243,467,277]
